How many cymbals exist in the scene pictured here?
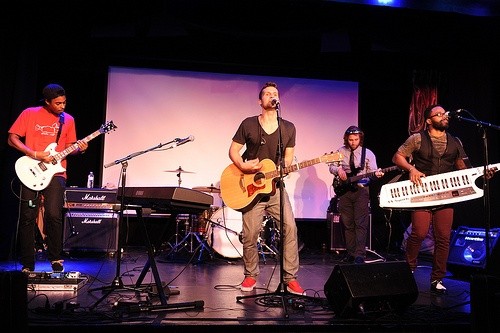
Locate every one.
[163,169,196,174]
[191,186,221,194]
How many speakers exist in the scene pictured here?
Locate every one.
[323,259,420,317]
[62,212,119,254]
[64,188,122,210]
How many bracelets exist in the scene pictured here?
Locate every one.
[35,151,37,160]
[409,167,415,172]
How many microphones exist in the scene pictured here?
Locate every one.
[269,98,281,107]
[170,135,195,151]
[444,107,462,118]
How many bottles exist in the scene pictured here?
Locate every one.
[87,172,94,188]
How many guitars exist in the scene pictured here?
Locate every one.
[220,149,343,213]
[332,166,396,195]
[13,119,118,192]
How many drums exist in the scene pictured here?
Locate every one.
[187,205,260,258]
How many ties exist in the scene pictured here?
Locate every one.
[350,149,357,176]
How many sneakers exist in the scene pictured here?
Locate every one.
[241,278,257,291]
[287,280,307,295]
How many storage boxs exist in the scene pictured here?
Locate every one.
[63,212,119,258]
[327,212,372,255]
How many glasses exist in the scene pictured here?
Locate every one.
[428,112,448,119]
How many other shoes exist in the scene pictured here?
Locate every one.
[22,268,35,272]
[343,256,364,265]
[431,281,446,291]
[51,260,65,272]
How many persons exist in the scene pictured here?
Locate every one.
[329,126,385,265]
[229,82,307,297]
[392,105,496,296]
[8,84,89,275]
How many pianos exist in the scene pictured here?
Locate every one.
[105,185,214,308]
[378,163,500,208]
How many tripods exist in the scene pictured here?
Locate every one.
[236,146,326,320]
[239,235,282,265]
[165,220,215,262]
[89,167,169,312]
